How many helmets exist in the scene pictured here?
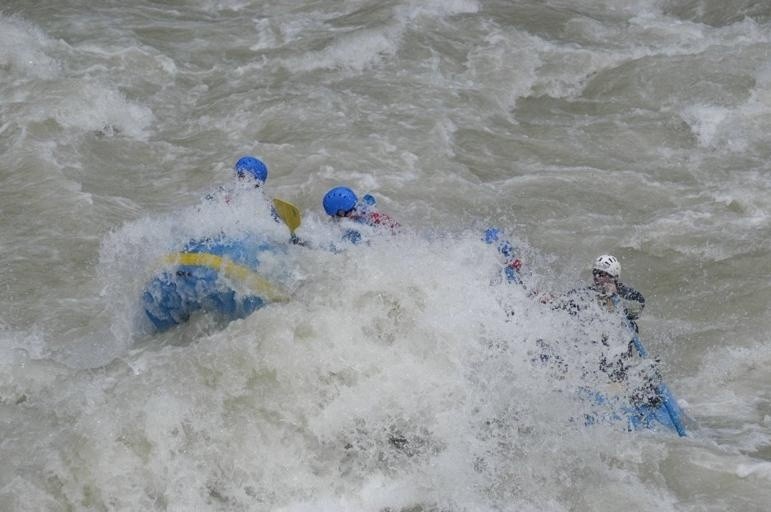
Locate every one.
[235,155,268,183]
[322,186,359,216]
[591,255,621,279]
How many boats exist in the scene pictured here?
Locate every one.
[144,241,679,431]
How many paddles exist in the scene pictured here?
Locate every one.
[272,193,302,246]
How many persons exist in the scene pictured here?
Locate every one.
[538,253,646,412]
[285,185,413,255]
[196,157,283,223]
